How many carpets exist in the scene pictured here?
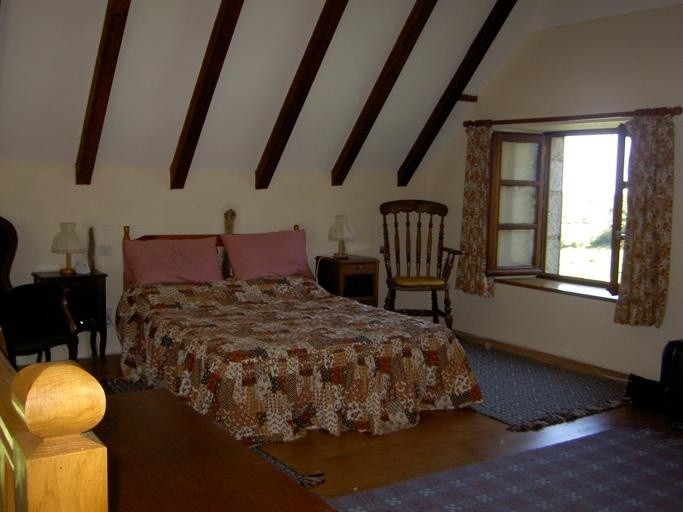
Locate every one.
[327,422,683,512]
[250,447,329,489]
[453,331,633,432]
[98,374,158,395]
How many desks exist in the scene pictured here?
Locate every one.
[92,386,337,512]
[31,267,108,361]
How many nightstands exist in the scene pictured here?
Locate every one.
[314,255,381,307]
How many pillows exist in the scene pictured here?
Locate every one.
[220,229,315,282]
[123,237,225,289]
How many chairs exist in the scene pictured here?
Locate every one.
[379,199,462,329]
[0,216,79,371]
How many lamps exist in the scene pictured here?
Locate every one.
[51,222,85,274]
[328,214,357,259]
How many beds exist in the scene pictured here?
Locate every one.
[115,222,484,444]
